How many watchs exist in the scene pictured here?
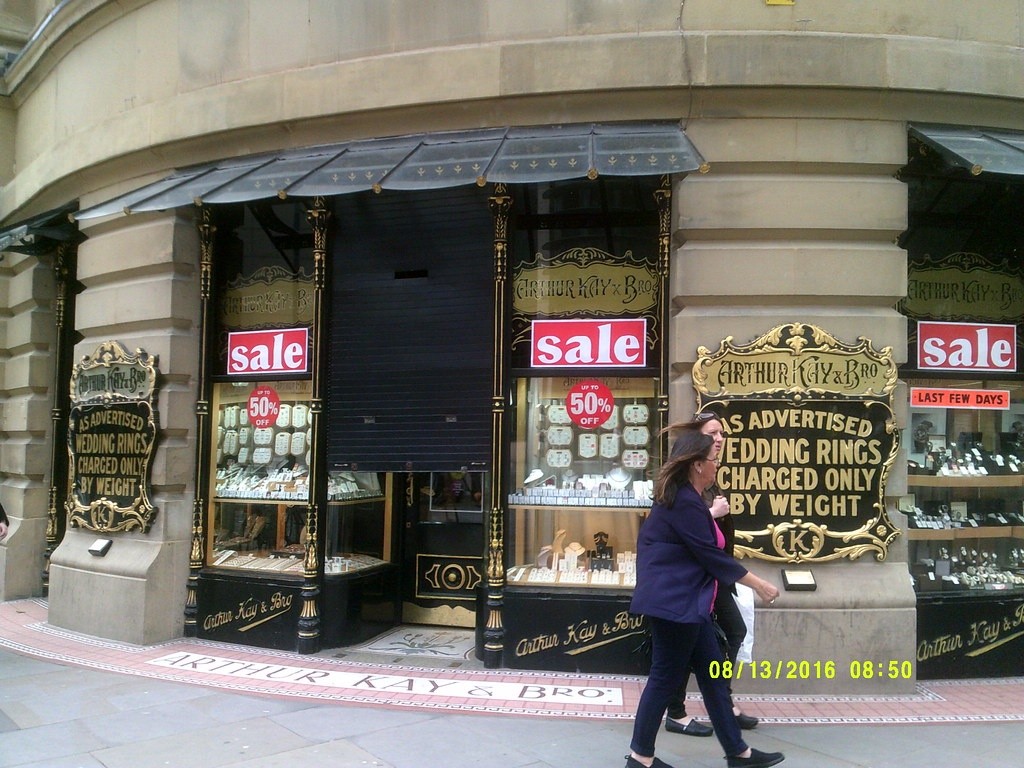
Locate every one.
[908,420,1024,475]
[921,547,1024,586]
[905,504,1024,529]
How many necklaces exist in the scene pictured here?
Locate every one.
[292,468,306,474]
[568,546,583,553]
[609,470,631,482]
[564,472,578,478]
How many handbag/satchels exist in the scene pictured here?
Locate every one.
[731,580,755,664]
[633,612,732,673]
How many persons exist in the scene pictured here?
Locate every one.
[0,502,9,542]
[624,412,785,768]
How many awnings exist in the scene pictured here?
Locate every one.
[908,123,1024,175]
[69,120,711,223]
[0,212,74,257]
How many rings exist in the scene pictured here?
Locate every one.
[770,600,774,603]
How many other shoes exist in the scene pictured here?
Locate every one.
[723,747,785,768]
[624,753,674,768]
[664,716,713,736]
[735,711,759,729]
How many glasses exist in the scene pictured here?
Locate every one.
[695,412,720,420]
[706,457,720,467]
